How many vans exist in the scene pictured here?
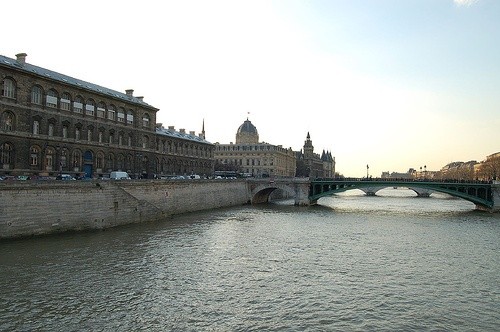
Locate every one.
[110,171,130,181]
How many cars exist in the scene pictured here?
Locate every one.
[3,174,111,181]
[160,174,237,180]
